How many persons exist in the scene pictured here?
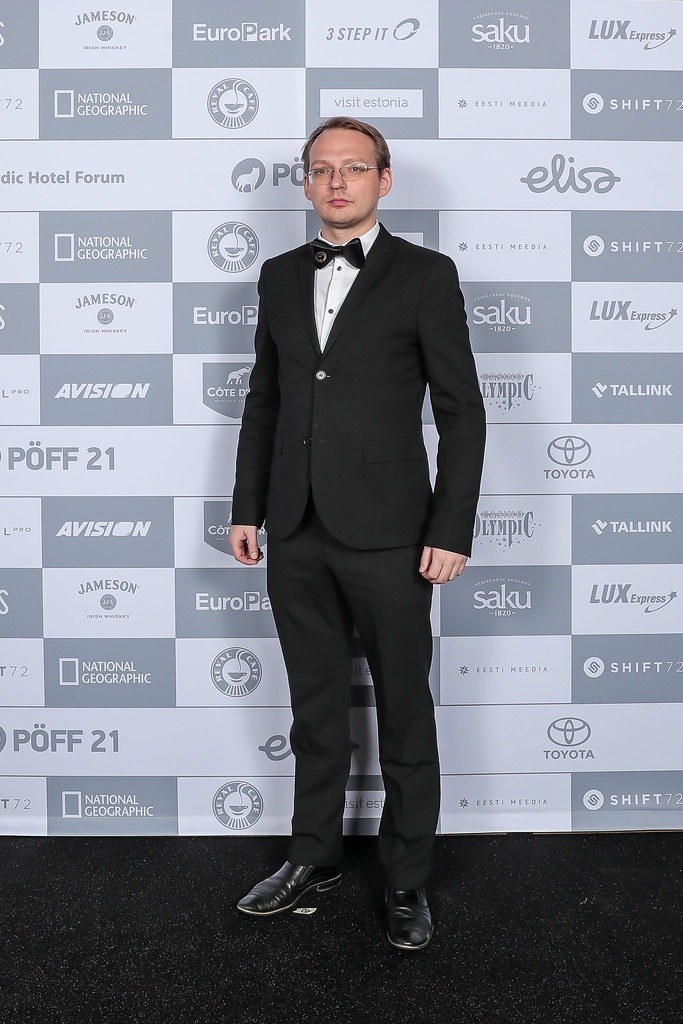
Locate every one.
[230,117,486,951]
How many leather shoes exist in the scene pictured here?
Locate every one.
[237,860,341,916]
[385,885,434,951]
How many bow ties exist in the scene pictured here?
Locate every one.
[309,238,366,270]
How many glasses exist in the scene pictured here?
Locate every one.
[305,163,380,186]
